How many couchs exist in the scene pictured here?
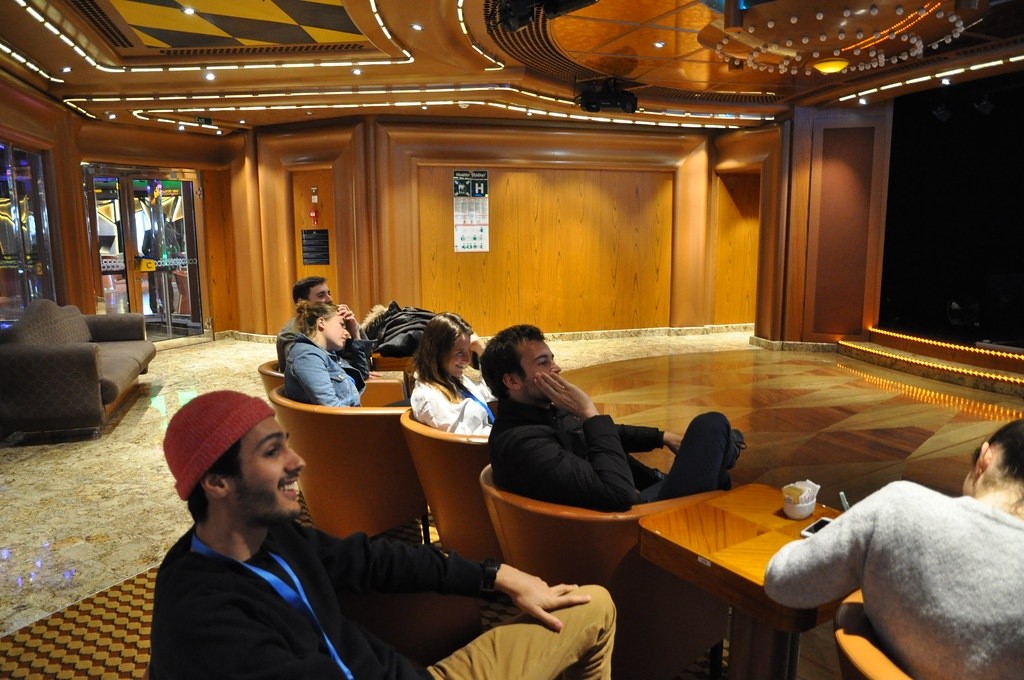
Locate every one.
[0,299,157,435]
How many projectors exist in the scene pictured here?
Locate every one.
[581,89,638,113]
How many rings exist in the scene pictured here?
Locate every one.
[353,314,355,317]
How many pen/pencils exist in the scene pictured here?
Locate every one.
[840,492,849,510]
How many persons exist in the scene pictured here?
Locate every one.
[276,276,373,373]
[405,311,497,438]
[479,324,748,513]
[148,390,618,680]
[763,418,1024,679]
[284,297,373,408]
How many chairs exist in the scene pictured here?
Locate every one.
[833,588,914,680]
[257,353,729,680]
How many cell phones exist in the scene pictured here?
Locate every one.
[800,516,834,538]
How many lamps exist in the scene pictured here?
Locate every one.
[714,5,964,75]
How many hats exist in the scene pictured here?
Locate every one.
[163,389,276,501]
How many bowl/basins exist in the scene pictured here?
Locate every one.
[783,498,816,519]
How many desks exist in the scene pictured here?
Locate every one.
[638,482,861,680]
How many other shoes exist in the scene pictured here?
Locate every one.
[641,469,669,492]
[729,430,748,468]
